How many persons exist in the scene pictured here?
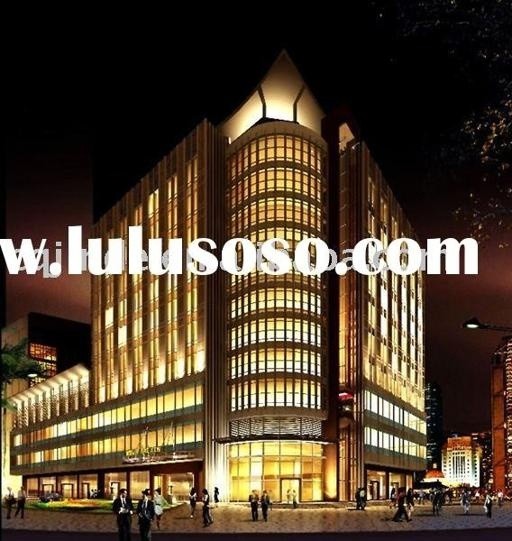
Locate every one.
[353,484,504,522]
[214,487,219,503]
[268,490,274,511]
[39,490,60,502]
[293,490,297,508]
[260,491,269,521]
[5,487,15,520]
[248,490,259,521]
[90,489,103,499]
[201,488,211,527]
[137,493,155,540]
[153,490,163,529]
[112,489,134,541]
[13,486,26,518]
[287,489,291,503]
[189,488,197,518]
